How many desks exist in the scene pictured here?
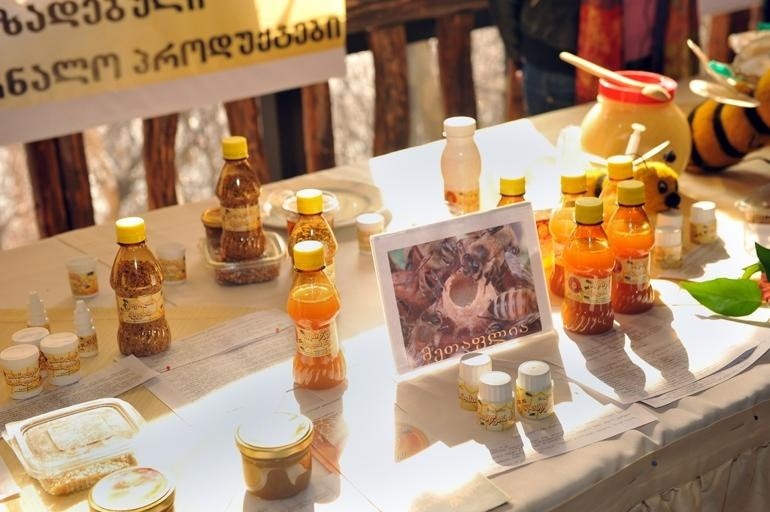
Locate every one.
[2,76,770,512]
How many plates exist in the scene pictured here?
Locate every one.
[258,178,384,231]
[734,182,770,250]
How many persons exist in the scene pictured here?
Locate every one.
[497,0,580,116]
[586,0,670,102]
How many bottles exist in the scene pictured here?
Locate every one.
[356,213,383,257]
[158,242,188,284]
[2,287,99,399]
[500,156,720,334]
[108,215,174,356]
[201,136,347,388]
[440,117,482,217]
[455,350,557,430]
[70,259,100,298]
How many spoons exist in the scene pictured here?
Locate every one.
[558,49,671,102]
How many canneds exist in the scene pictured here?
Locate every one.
[234,410,314,500]
[88,466,175,512]
